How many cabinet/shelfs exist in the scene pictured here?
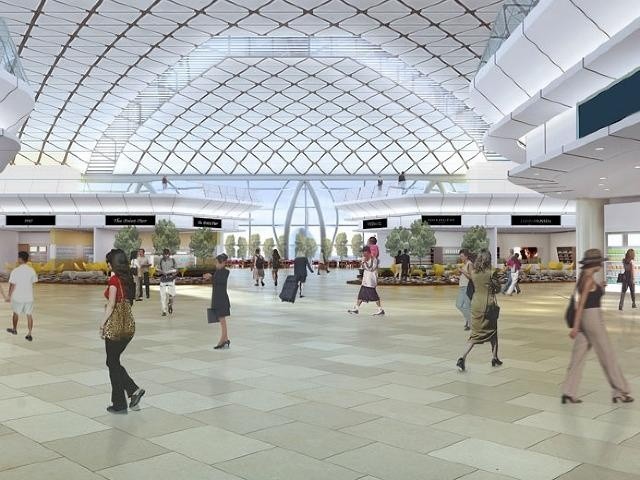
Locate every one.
[603,230,640,284]
[557,246,576,270]
